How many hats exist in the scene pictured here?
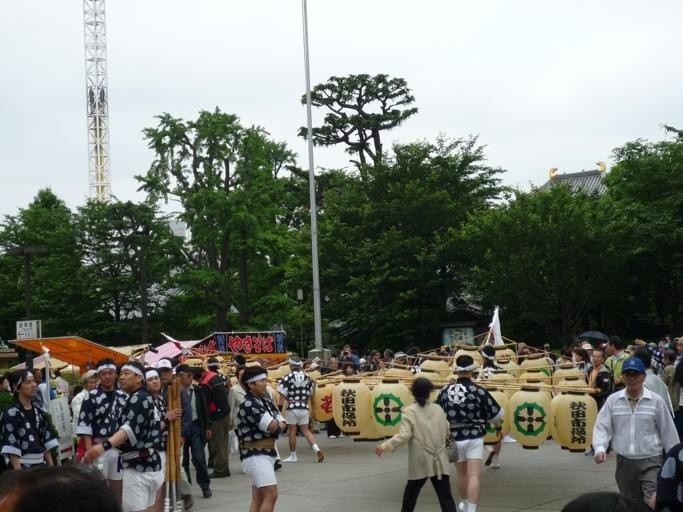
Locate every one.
[156,359,172,369]
[622,356,645,373]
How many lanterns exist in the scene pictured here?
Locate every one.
[453,346,599,452]
[271,359,453,442]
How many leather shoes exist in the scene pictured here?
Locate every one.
[202,487,212,497]
[208,470,230,478]
[184,495,192,509]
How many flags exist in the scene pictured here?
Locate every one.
[164,334,192,354]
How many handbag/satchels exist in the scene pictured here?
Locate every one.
[446,438,459,463]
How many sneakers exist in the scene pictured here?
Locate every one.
[485,449,495,466]
[282,456,297,462]
[315,449,323,462]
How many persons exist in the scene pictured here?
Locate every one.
[1,354,343,511]
[557,333,683,512]
[339,344,452,376]
[376,344,504,512]
